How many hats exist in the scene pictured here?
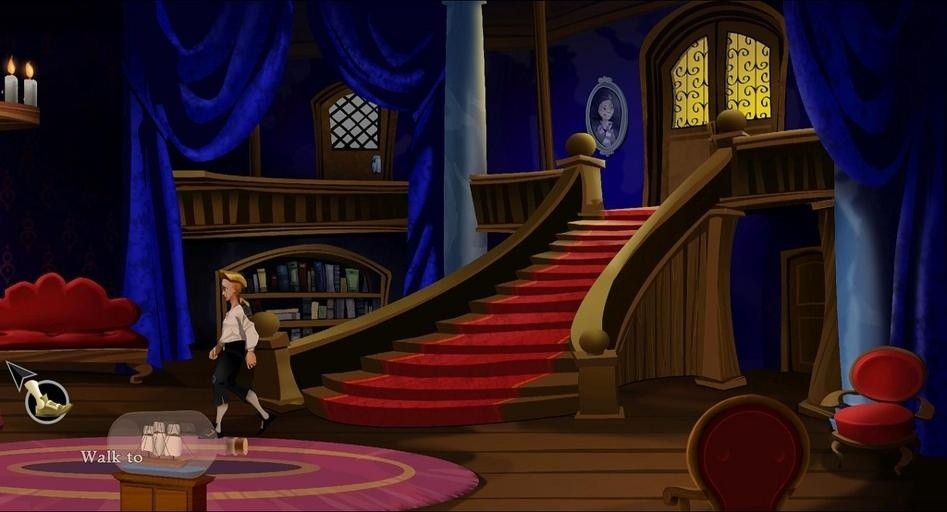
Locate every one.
[218,269,248,287]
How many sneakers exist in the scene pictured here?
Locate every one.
[256,413,276,433]
[35,393,74,419]
[212,424,224,437]
